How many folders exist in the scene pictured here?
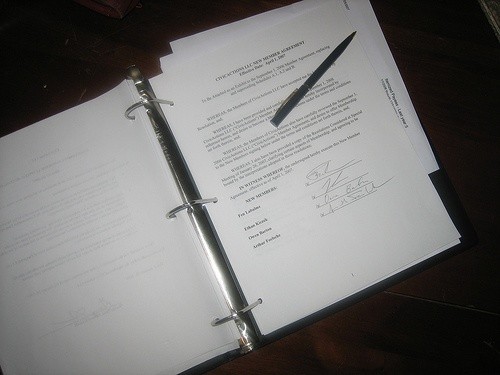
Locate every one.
[1,64,462,375]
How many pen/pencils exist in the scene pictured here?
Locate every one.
[271,30,358,134]
